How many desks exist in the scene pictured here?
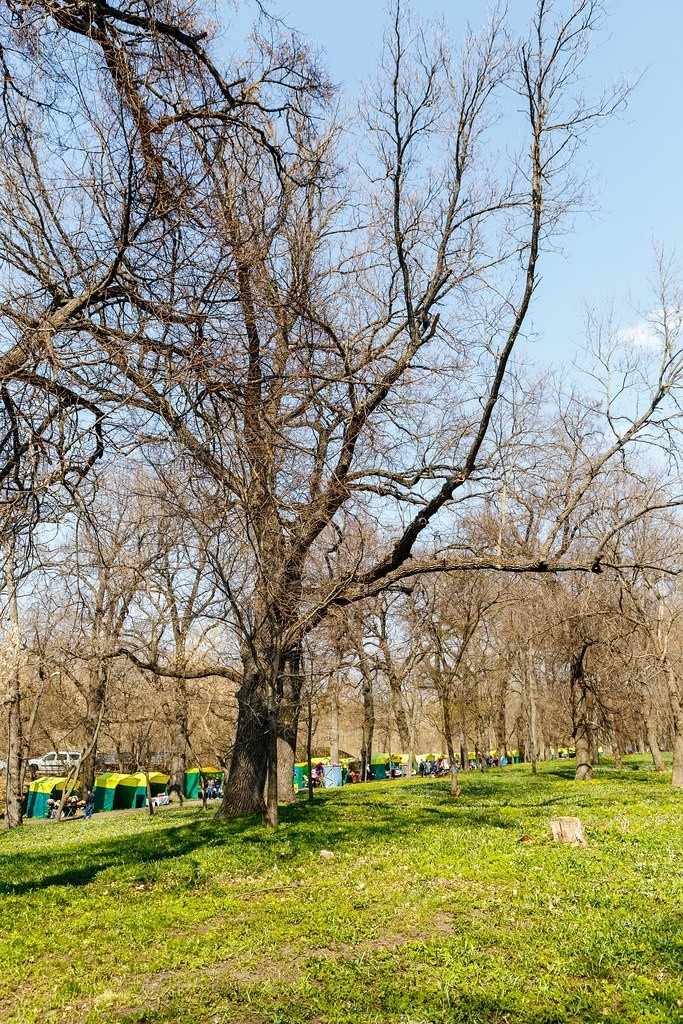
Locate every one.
[62,805,85,817]
[145,796,170,805]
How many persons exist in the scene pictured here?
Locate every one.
[81,754,508,820]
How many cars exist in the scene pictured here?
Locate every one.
[0,758,9,772]
[149,750,175,772]
[95,751,138,775]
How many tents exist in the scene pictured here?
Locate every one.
[183,766,225,798]
[91,773,139,809]
[294,745,603,818]
[131,772,170,798]
[26,775,88,820]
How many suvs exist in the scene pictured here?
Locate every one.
[28,750,81,773]
